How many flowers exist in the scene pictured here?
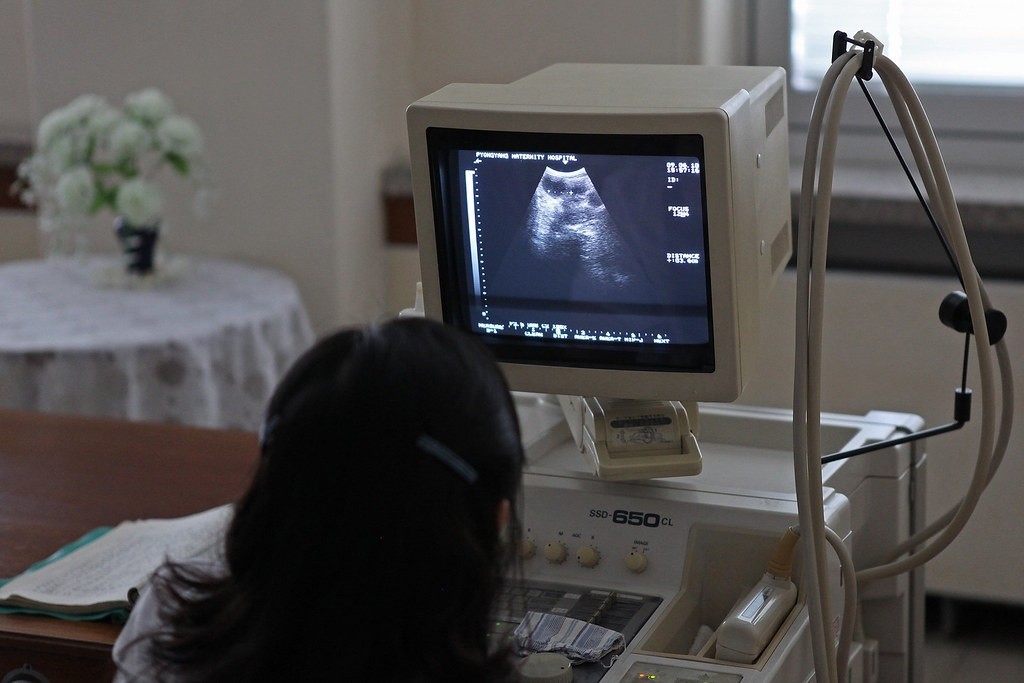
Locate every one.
[13,85,202,228]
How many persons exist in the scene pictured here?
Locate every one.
[111,316,526,683]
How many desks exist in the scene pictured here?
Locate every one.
[1,256,314,435]
[0,409,261,683]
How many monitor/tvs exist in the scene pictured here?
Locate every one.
[405,62,792,483]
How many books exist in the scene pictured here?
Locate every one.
[0,502,239,621]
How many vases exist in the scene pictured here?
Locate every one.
[112,209,160,273]
